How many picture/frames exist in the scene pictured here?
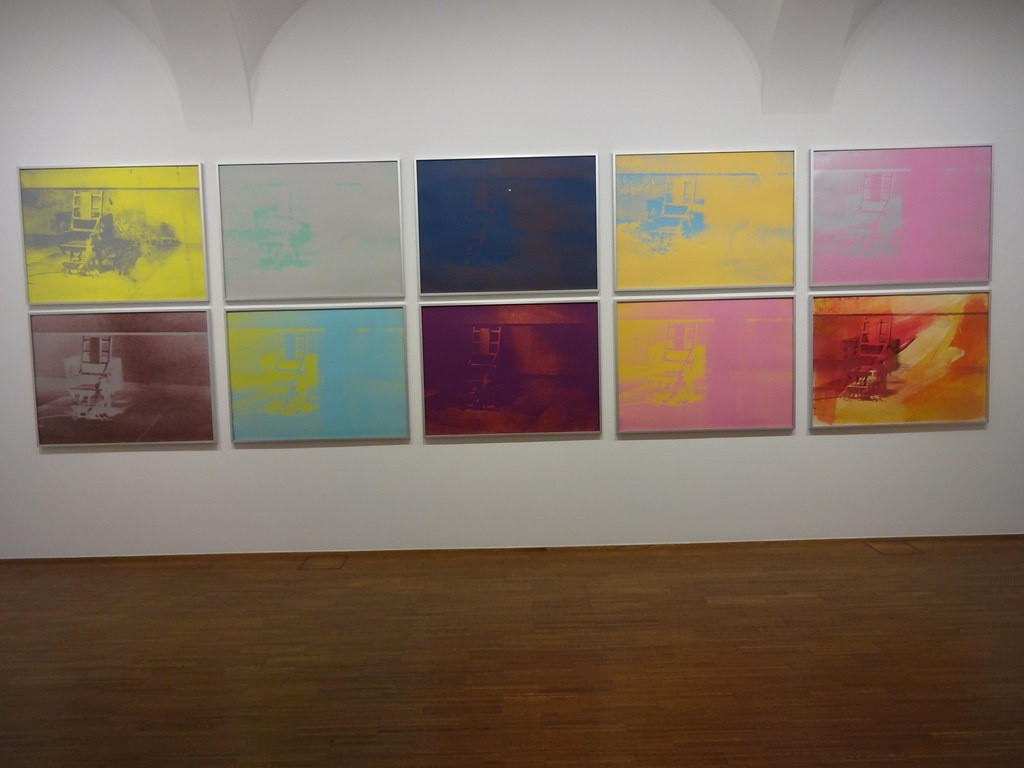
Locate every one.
[414,153,600,295]
[26,308,218,448]
[419,299,600,445]
[15,160,211,306]
[226,302,410,445]
[807,291,994,429]
[614,295,797,439]
[217,158,406,309]
[808,143,993,288]
[613,150,797,291]
